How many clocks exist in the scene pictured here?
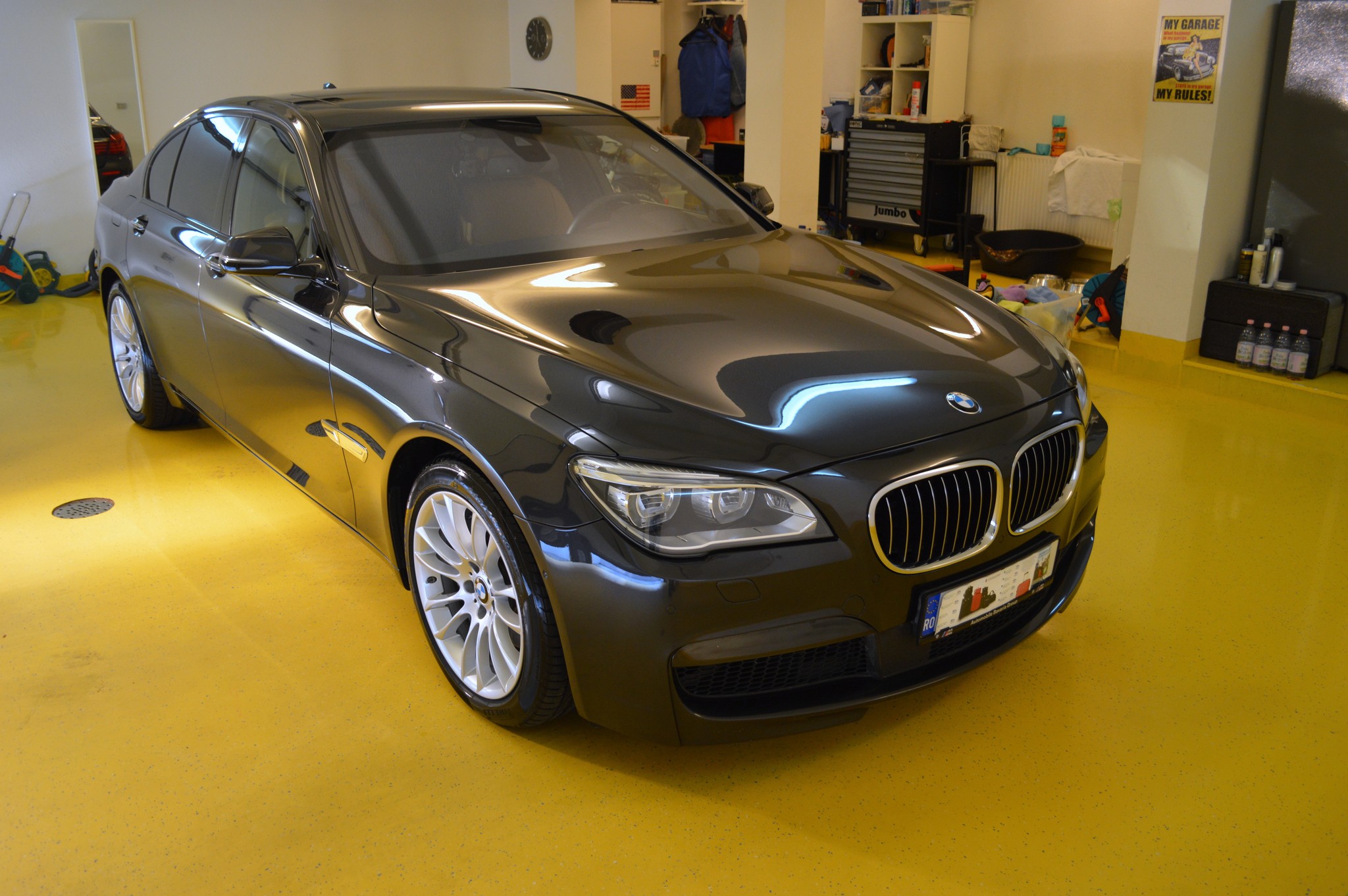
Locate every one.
[526,18,552,61]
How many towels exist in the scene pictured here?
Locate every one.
[1045,142,1139,222]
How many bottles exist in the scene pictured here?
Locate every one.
[1235,319,1257,369]
[1285,329,1310,381]
[1269,326,1292,376]
[1262,228,1274,283]
[1237,242,1253,282]
[1249,244,1267,285]
[1265,233,1284,286]
[976,274,990,289]
[1251,323,1274,373]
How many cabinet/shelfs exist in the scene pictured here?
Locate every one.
[854,14,970,123]
[1199,277,1348,379]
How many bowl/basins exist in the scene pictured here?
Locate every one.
[976,229,1085,280]
[1065,279,1090,293]
[1027,274,1065,290]
[859,95,891,114]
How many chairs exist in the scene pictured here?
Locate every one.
[264,156,397,264]
[461,140,573,247]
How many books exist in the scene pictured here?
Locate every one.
[861,0,928,16]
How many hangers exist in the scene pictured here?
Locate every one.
[679,16,733,44]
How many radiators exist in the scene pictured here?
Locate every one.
[970,151,1120,248]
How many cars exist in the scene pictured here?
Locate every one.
[86,102,135,194]
[92,81,1111,735]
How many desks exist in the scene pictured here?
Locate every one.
[709,140,844,236]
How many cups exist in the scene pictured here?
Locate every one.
[831,137,844,151]
[1052,115,1065,127]
[1036,143,1050,155]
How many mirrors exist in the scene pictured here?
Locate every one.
[77,18,146,195]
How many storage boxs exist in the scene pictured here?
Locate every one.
[859,96,891,115]
[1016,283,1084,350]
[920,0,976,16]
